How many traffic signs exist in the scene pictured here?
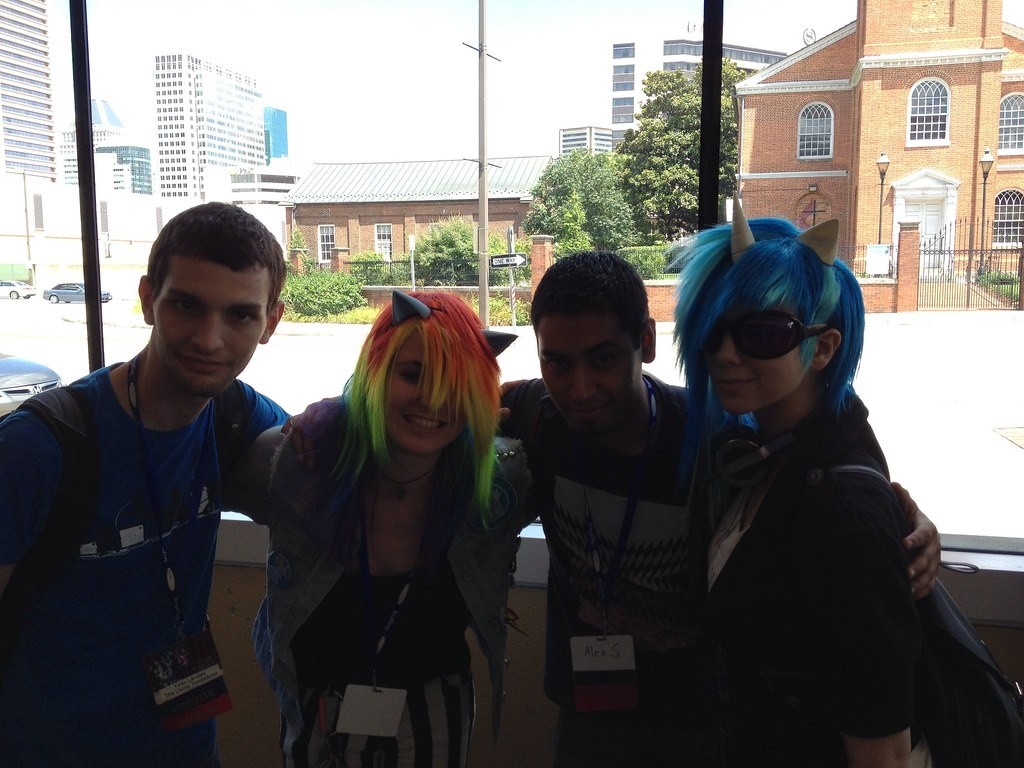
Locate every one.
[489,252,529,270]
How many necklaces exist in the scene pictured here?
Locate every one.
[378,455,439,500]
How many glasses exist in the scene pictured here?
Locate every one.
[701,309,830,359]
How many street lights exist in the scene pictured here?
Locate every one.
[874,151,891,245]
[976,147,997,276]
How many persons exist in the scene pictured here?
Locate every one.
[671,195,1024,768]
[0,201,530,768]
[220,287,539,768]
[293,248,939,767]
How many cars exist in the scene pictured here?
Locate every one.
[43,283,113,303]
[0,280,38,301]
[0,351,63,422]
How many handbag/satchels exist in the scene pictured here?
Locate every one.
[914,581,1024,767]
